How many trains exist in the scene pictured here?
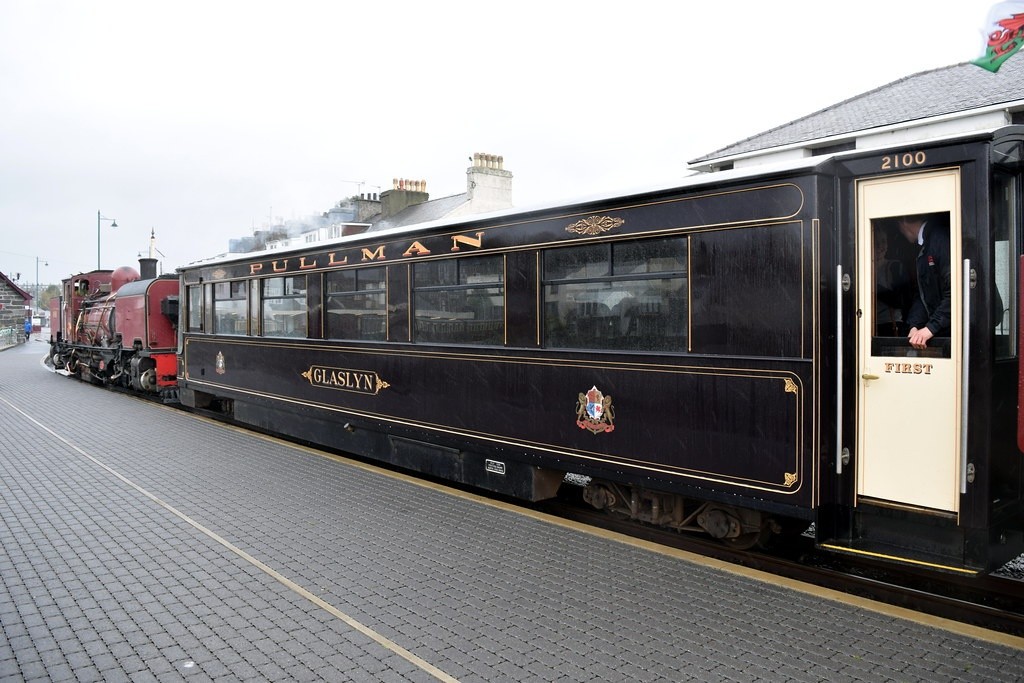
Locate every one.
[46,126,1023,627]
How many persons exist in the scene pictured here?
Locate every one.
[874,225,910,353]
[25,321,32,340]
[896,215,1004,365]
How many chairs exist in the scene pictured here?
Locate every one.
[547,294,675,349]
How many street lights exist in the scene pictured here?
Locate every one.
[138,249,152,260]
[36,260,48,316]
[97,217,119,272]
